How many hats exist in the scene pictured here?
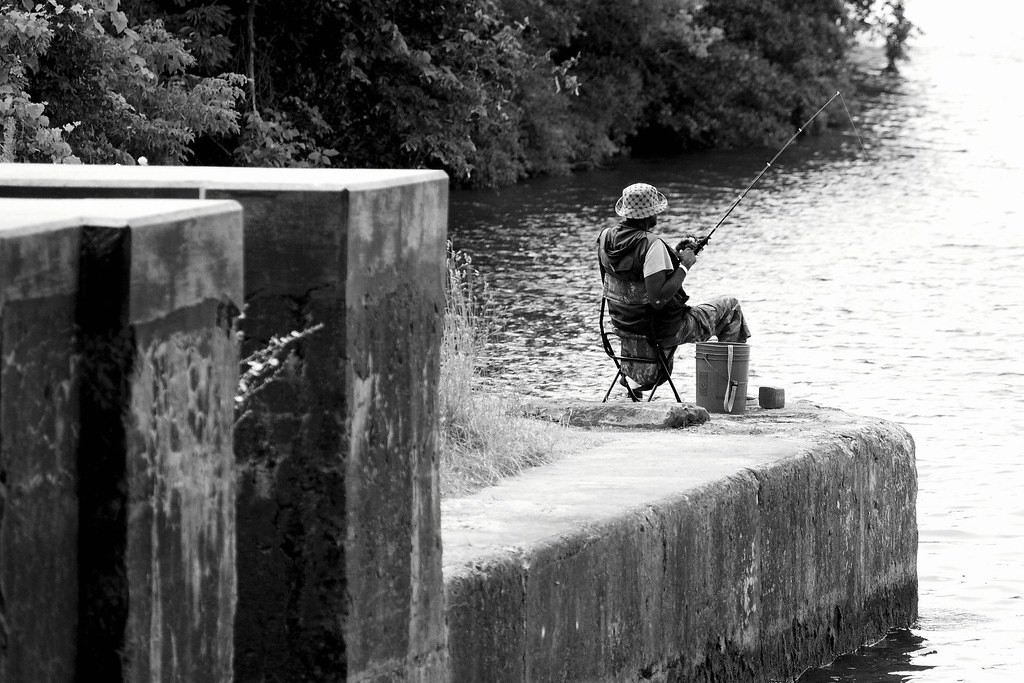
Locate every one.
[615,182,668,219]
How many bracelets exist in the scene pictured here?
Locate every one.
[679,264,688,274]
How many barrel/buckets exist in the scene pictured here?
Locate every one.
[695,342,752,414]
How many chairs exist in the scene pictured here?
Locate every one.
[600,273,685,403]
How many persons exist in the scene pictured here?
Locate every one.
[598,183,756,403]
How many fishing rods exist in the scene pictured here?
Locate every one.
[692,88,843,256]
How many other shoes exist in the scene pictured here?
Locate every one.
[626,390,643,402]
[746,393,756,399]
